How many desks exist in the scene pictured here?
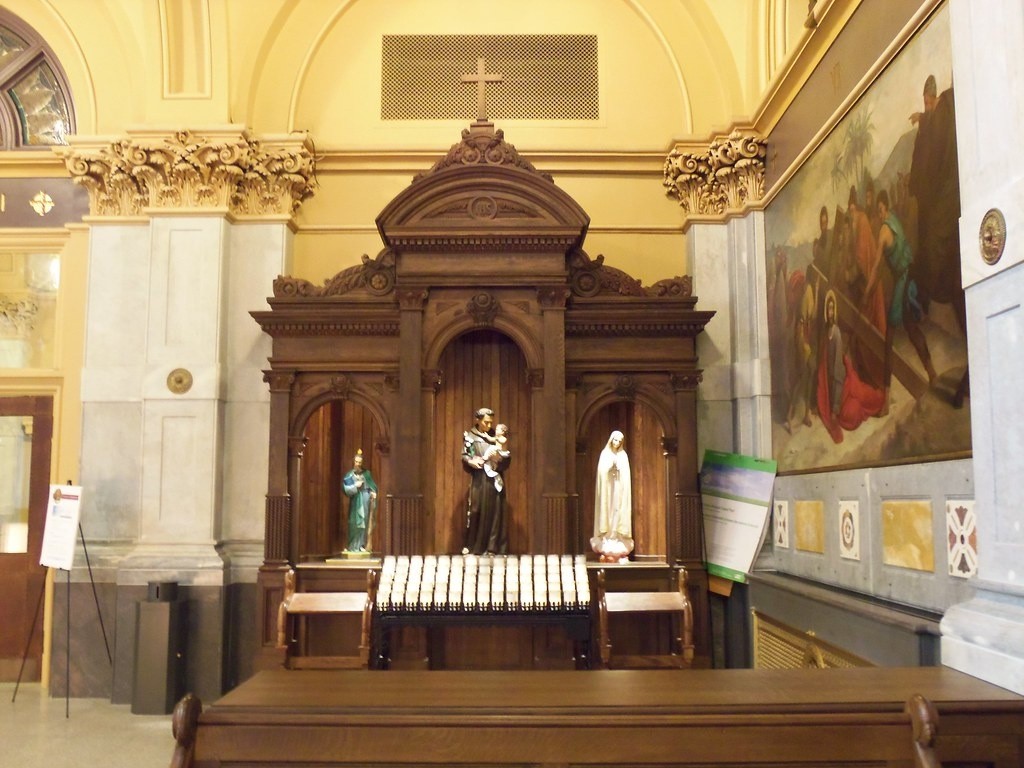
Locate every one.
[169,668,1024,768]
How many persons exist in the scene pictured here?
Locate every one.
[589,431,635,563]
[341,450,378,552]
[461,407,512,556]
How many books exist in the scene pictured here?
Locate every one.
[344,475,354,486]
